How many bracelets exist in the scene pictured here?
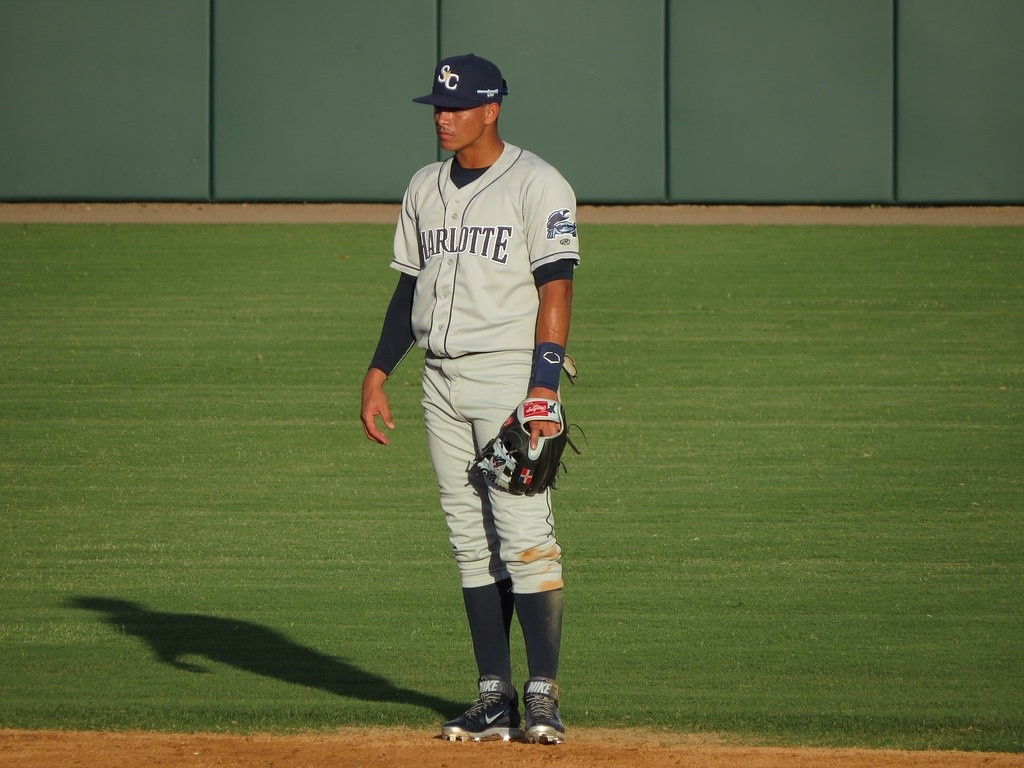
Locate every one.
[528,342,565,393]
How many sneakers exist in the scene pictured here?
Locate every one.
[441,676,523,741]
[523,677,565,744]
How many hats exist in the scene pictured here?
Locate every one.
[411,52,506,109]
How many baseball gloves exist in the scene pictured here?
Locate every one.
[473,397,568,497]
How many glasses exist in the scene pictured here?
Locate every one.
[474,77,509,96]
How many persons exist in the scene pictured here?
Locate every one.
[360,57,580,746]
